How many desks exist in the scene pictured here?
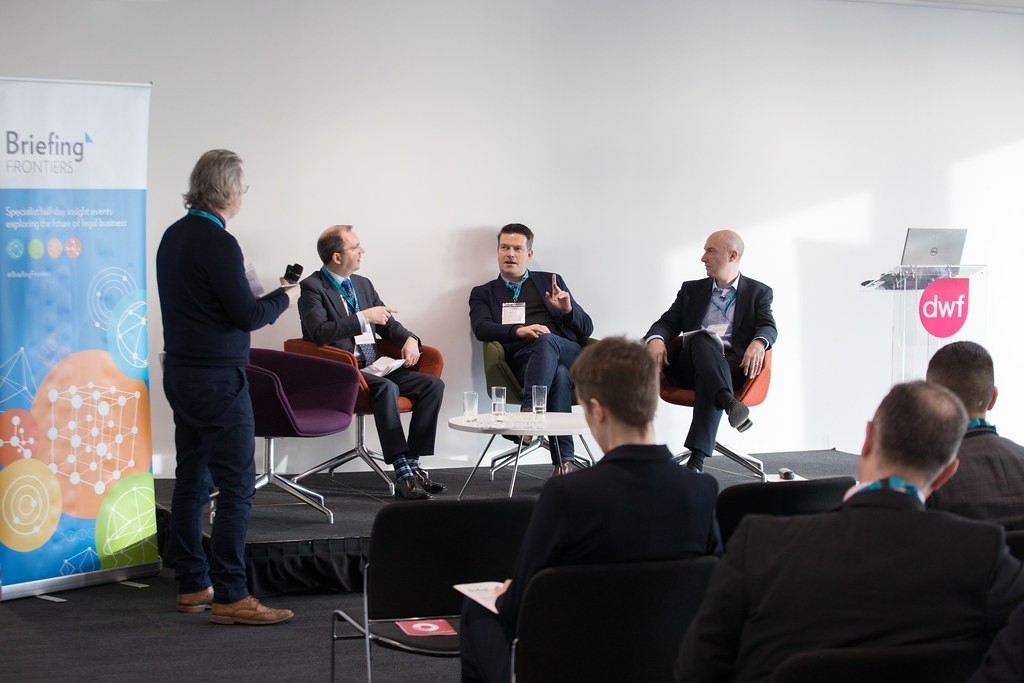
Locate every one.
[447,412,600,499]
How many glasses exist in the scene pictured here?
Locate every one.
[241,184,251,194]
[338,242,360,253]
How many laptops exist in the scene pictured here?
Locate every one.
[878,229,967,290]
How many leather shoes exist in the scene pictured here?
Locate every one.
[209,596,294,625]
[726,399,753,433]
[552,460,572,478]
[414,469,449,493]
[686,465,705,474]
[176,585,215,612]
[501,434,533,446]
[394,476,432,500]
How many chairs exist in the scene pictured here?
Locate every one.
[283,336,445,496]
[483,336,601,481]
[205,347,360,526]
[654,335,772,481]
[329,477,989,683]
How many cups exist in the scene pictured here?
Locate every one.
[532,385,548,414]
[464,391,479,422]
[491,386,506,416]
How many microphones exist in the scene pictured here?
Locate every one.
[269,263,303,325]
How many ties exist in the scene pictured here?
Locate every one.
[343,282,375,366]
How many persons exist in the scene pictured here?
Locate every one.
[640,230,778,473]
[675,341,1024,683]
[297,225,448,502]
[459,335,719,683]
[469,224,594,479]
[156,150,301,624]
